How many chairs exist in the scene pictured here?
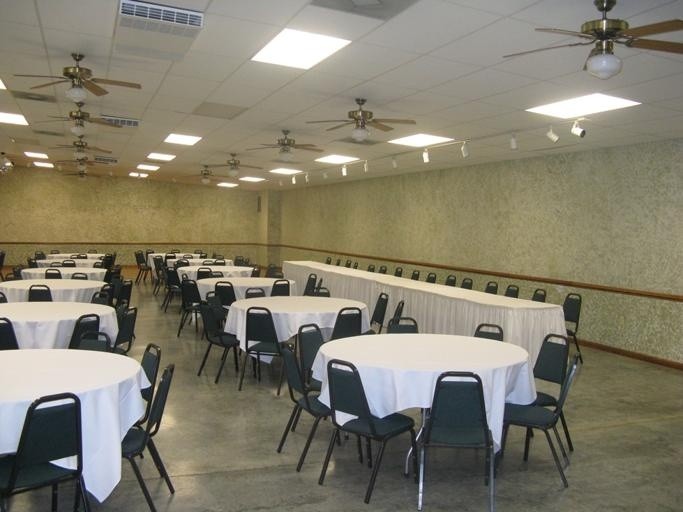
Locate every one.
[0,251,585,512]
[564,293,585,366]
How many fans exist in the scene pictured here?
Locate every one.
[178,97,416,187]
[501,0,682,81]
[10,52,142,183]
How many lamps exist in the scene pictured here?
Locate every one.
[584,41,624,82]
[276,120,586,188]
